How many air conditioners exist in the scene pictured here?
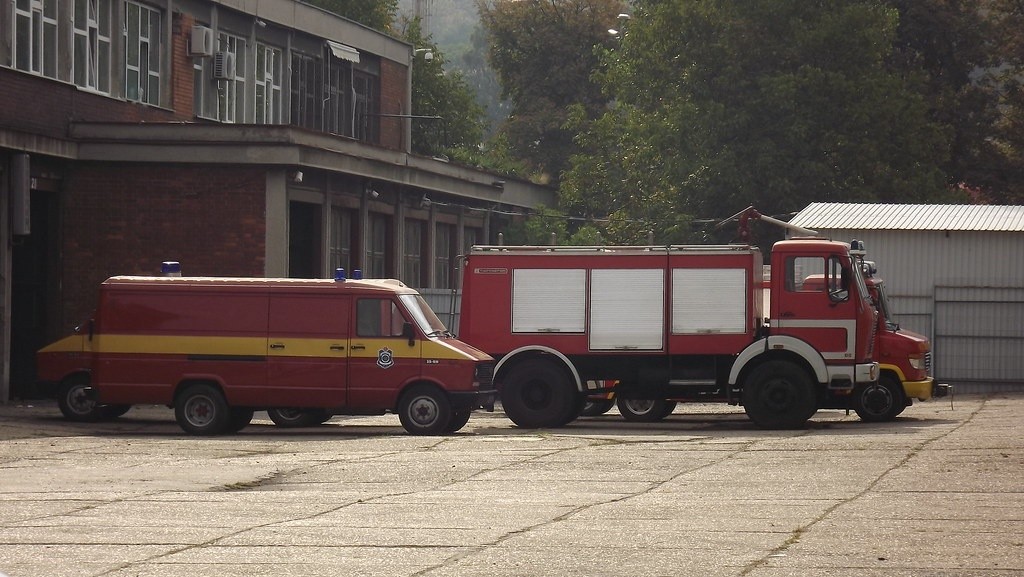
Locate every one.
[191,25,214,56]
[214,52,235,81]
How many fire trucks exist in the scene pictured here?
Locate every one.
[36,300,132,424]
[86,261,499,437]
[448,204,936,431]
